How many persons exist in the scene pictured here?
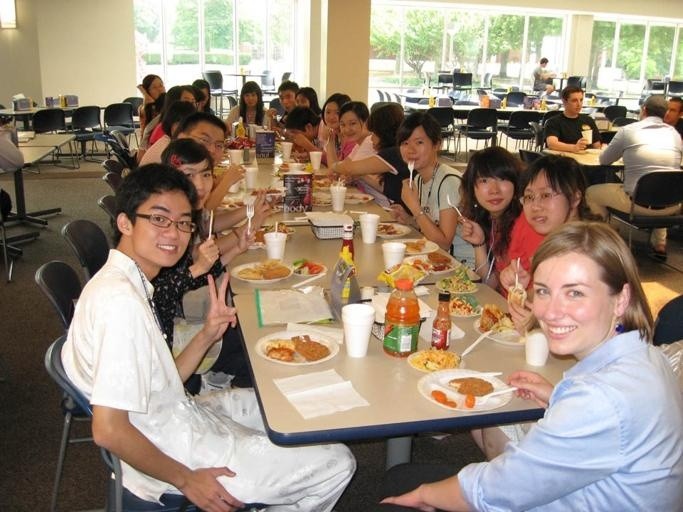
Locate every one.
[135,75,281,388]
[0,129,24,216]
[545,85,682,261]
[366,220,683,512]
[472,156,586,458]
[533,58,553,96]
[268,81,543,299]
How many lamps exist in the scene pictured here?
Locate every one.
[0,0,20,31]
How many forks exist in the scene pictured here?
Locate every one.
[446,195,465,221]
[406,161,415,190]
[245,202,254,234]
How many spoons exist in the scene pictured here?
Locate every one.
[511,257,523,294]
[473,386,522,405]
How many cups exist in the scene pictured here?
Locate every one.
[339,304,376,359]
[262,231,285,261]
[525,326,549,367]
[244,168,258,191]
[580,129,593,145]
[309,151,322,170]
[358,213,380,244]
[228,150,241,165]
[329,186,346,211]
[280,142,292,160]
[287,163,305,172]
[382,242,406,266]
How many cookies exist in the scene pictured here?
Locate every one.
[295,341,330,362]
[448,377,494,396]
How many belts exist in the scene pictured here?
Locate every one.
[626,193,681,210]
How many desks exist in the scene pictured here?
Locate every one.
[185,137,579,448]
[542,144,630,184]
[406,99,554,153]
[1,123,76,231]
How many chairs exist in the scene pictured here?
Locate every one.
[33,133,267,510]
[3,94,147,161]
[373,65,683,272]
[195,66,294,118]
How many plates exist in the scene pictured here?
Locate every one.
[307,177,374,205]
[255,327,338,366]
[449,305,483,317]
[474,317,527,347]
[258,226,296,234]
[417,368,514,412]
[407,351,466,374]
[289,264,327,277]
[387,238,439,254]
[405,255,461,275]
[230,261,293,287]
[435,280,478,294]
[375,223,411,239]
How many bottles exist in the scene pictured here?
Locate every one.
[329,226,372,321]
[385,280,422,358]
[243,147,249,162]
[235,117,245,137]
[247,125,256,139]
[430,290,452,349]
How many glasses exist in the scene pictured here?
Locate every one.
[519,190,561,205]
[132,212,197,233]
[189,134,226,152]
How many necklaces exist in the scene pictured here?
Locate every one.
[59,163,358,511]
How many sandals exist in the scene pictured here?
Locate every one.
[650,248,667,262]
